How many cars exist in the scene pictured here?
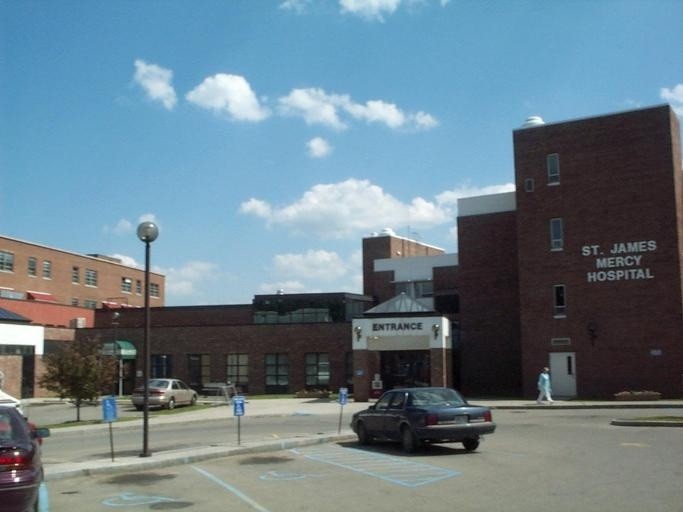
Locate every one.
[131,377,199,410]
[348,386,498,452]
[0,405,52,510]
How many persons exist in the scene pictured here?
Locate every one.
[535,366,555,404]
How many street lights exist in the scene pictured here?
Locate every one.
[136,221,159,457]
